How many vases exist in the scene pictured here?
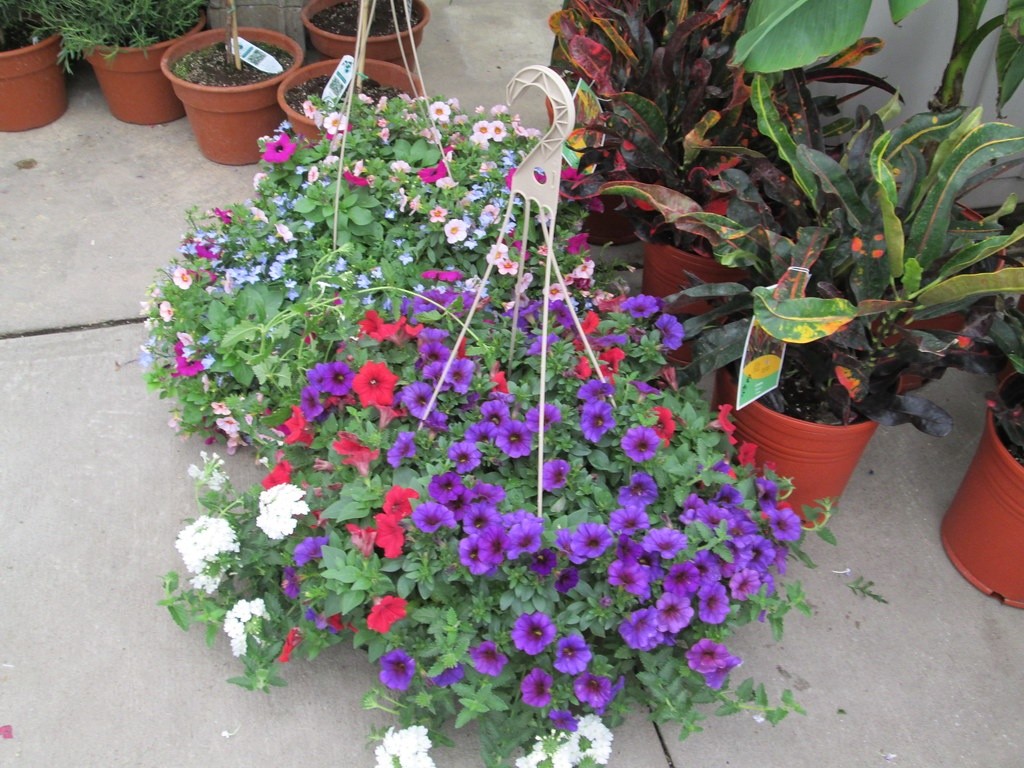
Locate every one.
[161,25,304,166]
[300,0,431,73]
[275,58,424,142]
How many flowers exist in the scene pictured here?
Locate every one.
[137,84,889,768]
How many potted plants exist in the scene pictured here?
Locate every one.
[549,0,1024,611]
[0,0,68,133]
[19,0,207,124]
